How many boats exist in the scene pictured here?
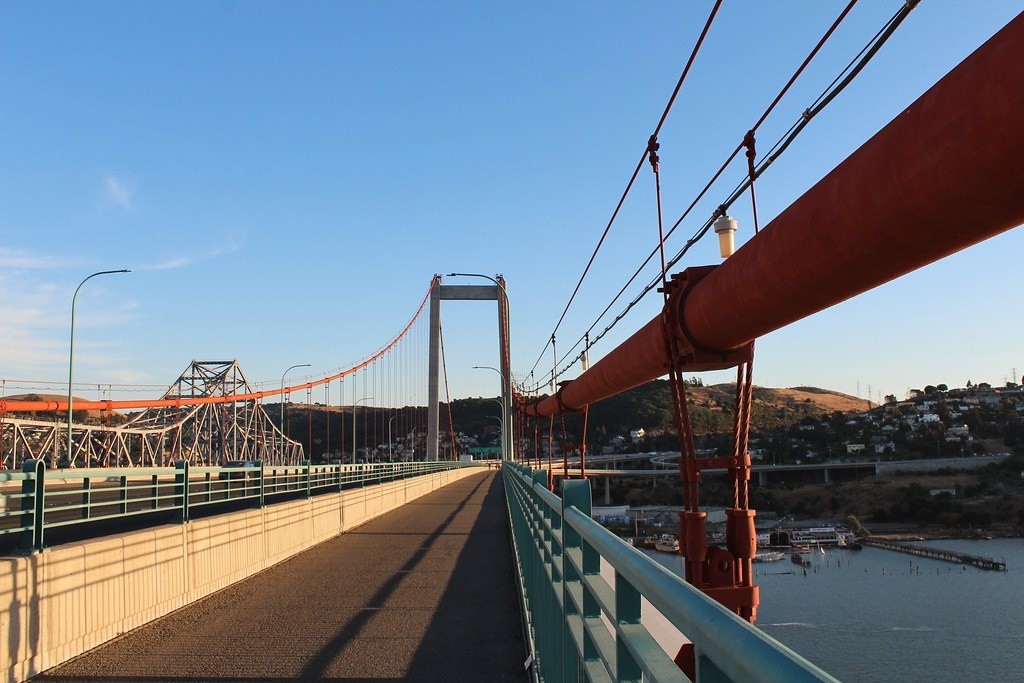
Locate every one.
[753,550,785,562]
[789,552,812,567]
[655,536,681,553]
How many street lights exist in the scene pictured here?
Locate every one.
[352,397,374,465]
[472,366,508,461]
[389,414,405,461]
[280,365,312,465]
[64,270,131,467]
[447,272,516,462]
[412,425,426,460]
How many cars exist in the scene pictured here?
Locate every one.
[217,460,256,483]
